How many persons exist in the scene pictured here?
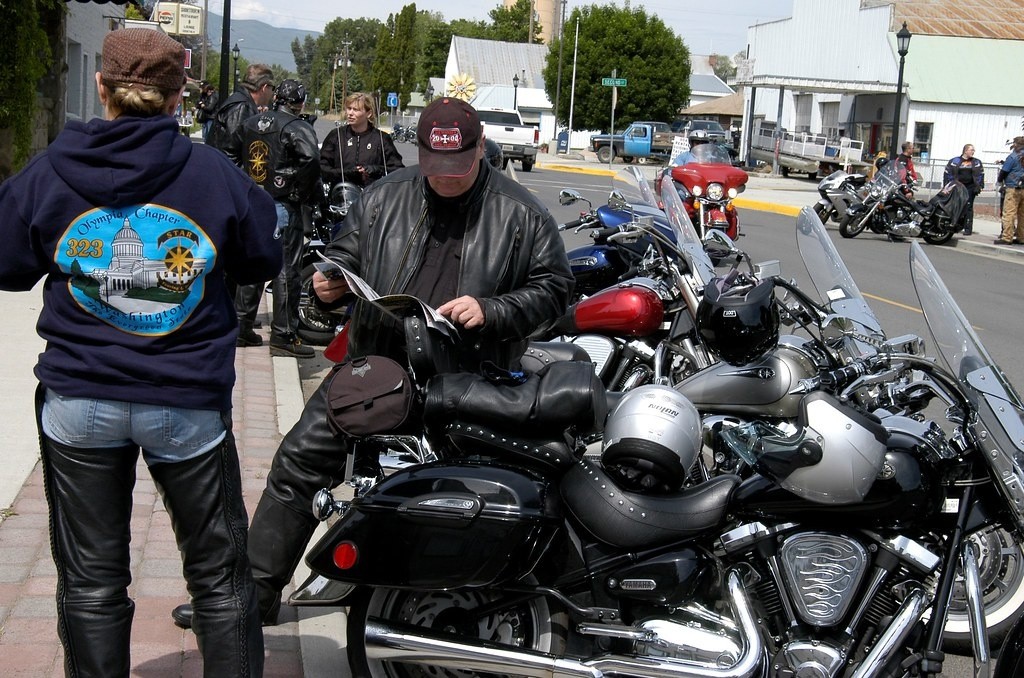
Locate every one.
[894,142,917,200]
[944,144,984,235]
[0,28,281,678]
[206,64,276,328]
[170,94,575,627]
[222,78,321,358]
[994,137,1024,246]
[191,80,219,141]
[671,130,709,199]
[319,92,406,186]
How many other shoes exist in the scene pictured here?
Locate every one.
[1012,239,1023,244]
[994,239,1011,245]
[963,229,971,235]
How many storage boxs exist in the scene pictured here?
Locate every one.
[304,460,550,595]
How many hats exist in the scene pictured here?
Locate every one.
[198,80,208,88]
[102,28,187,90]
[417,96,481,177]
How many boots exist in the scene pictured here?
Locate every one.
[170,492,320,627]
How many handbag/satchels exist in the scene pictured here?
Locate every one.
[197,108,206,123]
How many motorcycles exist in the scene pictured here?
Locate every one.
[389,121,419,146]
[808,166,890,235]
[837,159,969,246]
[283,162,1024,678]
[651,143,749,267]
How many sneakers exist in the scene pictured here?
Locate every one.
[236,331,262,347]
[269,341,315,359]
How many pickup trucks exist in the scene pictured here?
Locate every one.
[473,106,540,171]
[587,124,673,164]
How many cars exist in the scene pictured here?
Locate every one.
[678,120,725,139]
[633,120,672,133]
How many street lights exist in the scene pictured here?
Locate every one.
[232,43,241,96]
[511,73,520,110]
[428,86,435,103]
[889,20,915,160]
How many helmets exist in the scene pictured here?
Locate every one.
[688,130,710,143]
[875,158,889,169]
[329,183,361,214]
[694,274,780,365]
[484,138,503,169]
[600,385,704,492]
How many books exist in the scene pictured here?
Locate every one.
[312,248,461,345]
